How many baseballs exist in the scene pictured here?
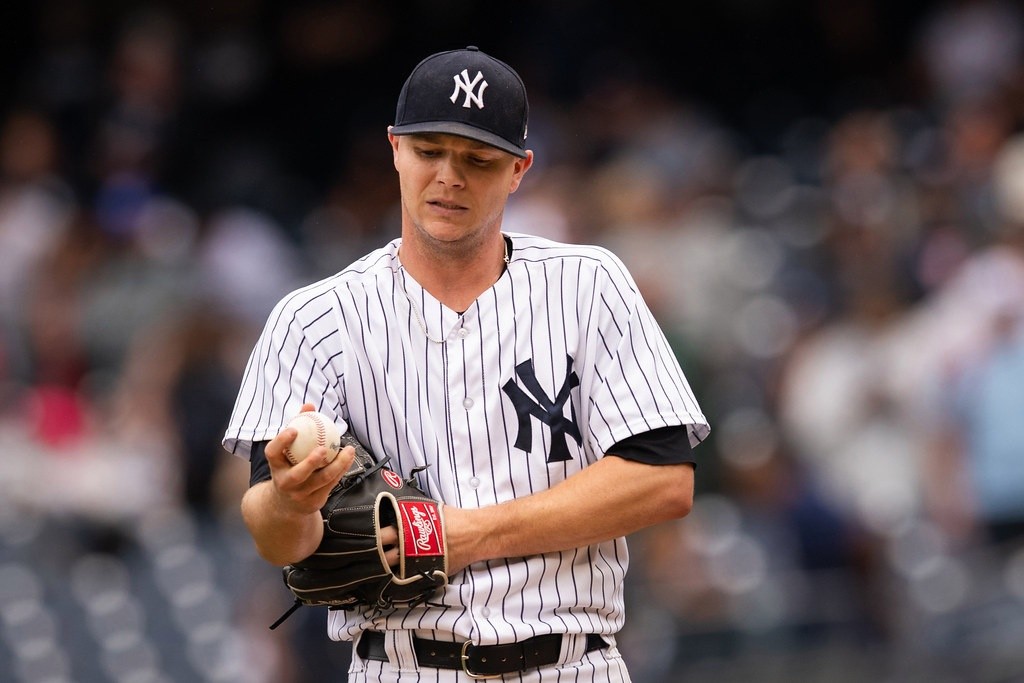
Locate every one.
[278,410,339,470]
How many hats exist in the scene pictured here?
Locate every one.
[390,46,530,159]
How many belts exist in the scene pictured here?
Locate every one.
[357,631,607,679]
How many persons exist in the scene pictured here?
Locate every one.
[0,0,1024,683]
[221,46,710,683]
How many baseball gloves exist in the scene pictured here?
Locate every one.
[282,430,448,614]
